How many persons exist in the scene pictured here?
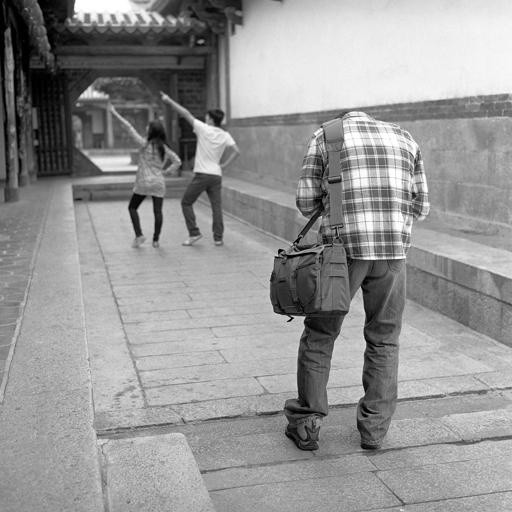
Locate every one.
[284,107,432,451]
[108,104,182,247]
[159,89,240,246]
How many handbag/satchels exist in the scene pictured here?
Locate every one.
[269,238,352,322]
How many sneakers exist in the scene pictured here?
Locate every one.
[131,235,148,248]
[152,241,160,247]
[183,234,203,246]
[284,416,323,452]
